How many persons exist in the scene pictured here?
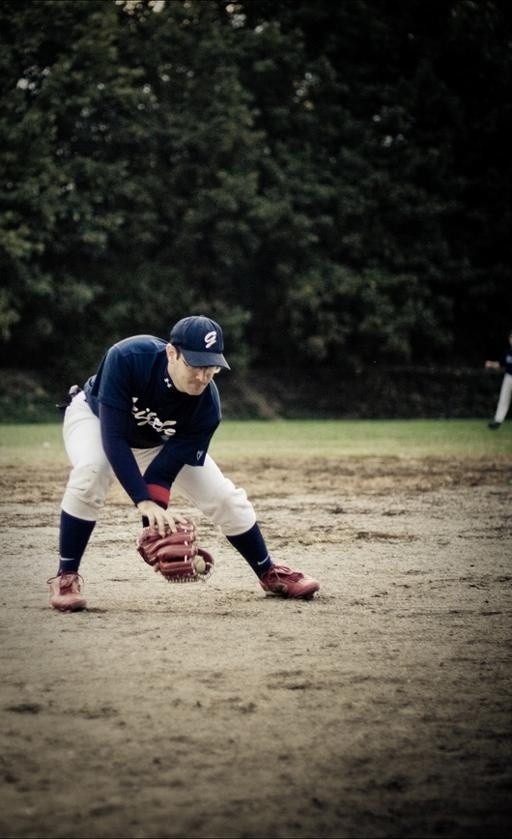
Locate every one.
[486,333,512,430]
[48,313,321,610]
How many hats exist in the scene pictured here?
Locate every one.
[169,315,232,372]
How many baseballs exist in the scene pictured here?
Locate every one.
[193,555,206,574]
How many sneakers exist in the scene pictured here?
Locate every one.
[47,573,87,611]
[260,562,320,599]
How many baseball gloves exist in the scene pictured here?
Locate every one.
[138,523,213,581]
